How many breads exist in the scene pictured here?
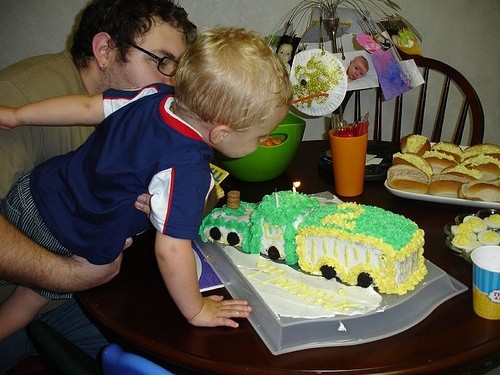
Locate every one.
[432,142,462,160]
[428,172,477,199]
[387,164,428,193]
[441,165,482,183]
[400,133,431,157]
[392,152,434,178]
[463,143,500,162]
[420,150,459,172]
[462,154,500,179]
[456,179,499,203]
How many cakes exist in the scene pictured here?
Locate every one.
[451,234,480,250]
[200,188,428,297]
[484,214,500,230]
[477,230,500,246]
[450,222,478,241]
[463,216,487,232]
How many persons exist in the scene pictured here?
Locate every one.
[391,31,414,48]
[372,33,393,50]
[342,56,369,82]
[1,0,198,375]
[1,24,294,343]
[277,43,294,78]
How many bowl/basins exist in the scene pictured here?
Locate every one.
[217,110,306,182]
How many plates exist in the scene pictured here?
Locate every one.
[289,48,348,116]
[444,208,499,263]
[384,142,500,210]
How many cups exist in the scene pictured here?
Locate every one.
[471,246,500,320]
[329,126,368,197]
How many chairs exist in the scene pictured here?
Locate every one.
[328,54,484,164]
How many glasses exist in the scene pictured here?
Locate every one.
[121,37,179,76]
